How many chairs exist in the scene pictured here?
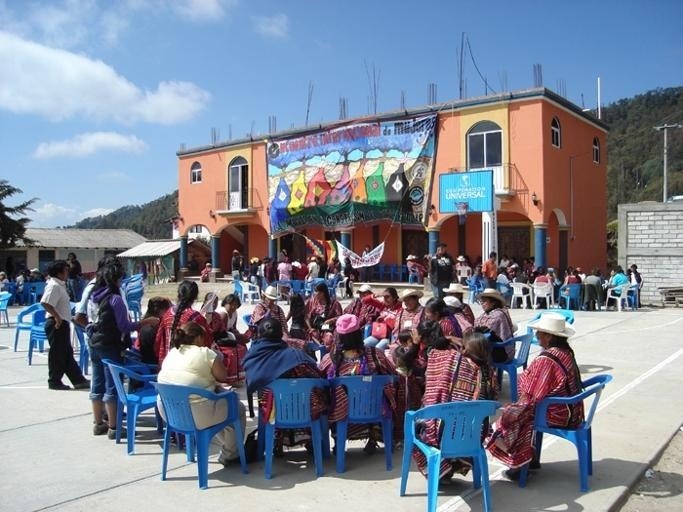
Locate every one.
[408,268,423,284]
[329,375,399,473]
[101,358,162,453]
[364,323,373,339]
[455,265,639,311]
[357,263,426,282]
[519,374,612,492]
[72,316,89,375]
[490,331,534,403]
[233,276,349,304]
[400,400,501,512]
[256,377,330,479]
[0,277,77,365]
[527,308,574,344]
[149,381,249,489]
[243,314,253,323]
[121,273,145,323]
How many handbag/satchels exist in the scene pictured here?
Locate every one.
[244,436,266,463]
[372,321,387,339]
[311,313,336,332]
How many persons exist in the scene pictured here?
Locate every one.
[412,333,501,482]
[84,264,161,439]
[1,243,650,377]
[329,314,396,456]
[240,320,329,457]
[39,260,91,390]
[156,321,246,466]
[399,319,449,403]
[484,312,585,479]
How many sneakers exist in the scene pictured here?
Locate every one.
[503,468,533,483]
[48,383,70,391]
[218,453,251,467]
[522,461,543,472]
[107,427,138,440]
[73,380,91,389]
[93,422,109,436]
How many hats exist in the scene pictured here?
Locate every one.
[456,255,466,262]
[476,287,506,307]
[441,282,468,294]
[355,284,373,294]
[398,288,424,303]
[442,295,463,309]
[336,313,360,335]
[526,310,577,338]
[406,254,416,260]
[261,284,279,301]
